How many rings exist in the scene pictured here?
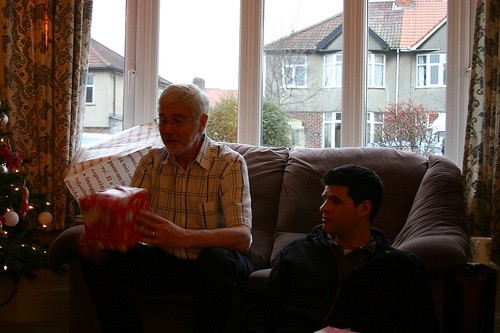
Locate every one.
[152,232,155,238]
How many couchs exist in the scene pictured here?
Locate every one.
[47,142,472,333]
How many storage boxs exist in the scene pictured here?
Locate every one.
[80,185,151,252]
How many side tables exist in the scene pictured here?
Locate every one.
[441,260,498,333]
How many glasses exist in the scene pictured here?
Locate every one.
[154,115,194,127]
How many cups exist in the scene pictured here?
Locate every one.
[470,236,493,265]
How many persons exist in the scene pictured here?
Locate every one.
[240,164,440,333]
[78,84,254,333]
